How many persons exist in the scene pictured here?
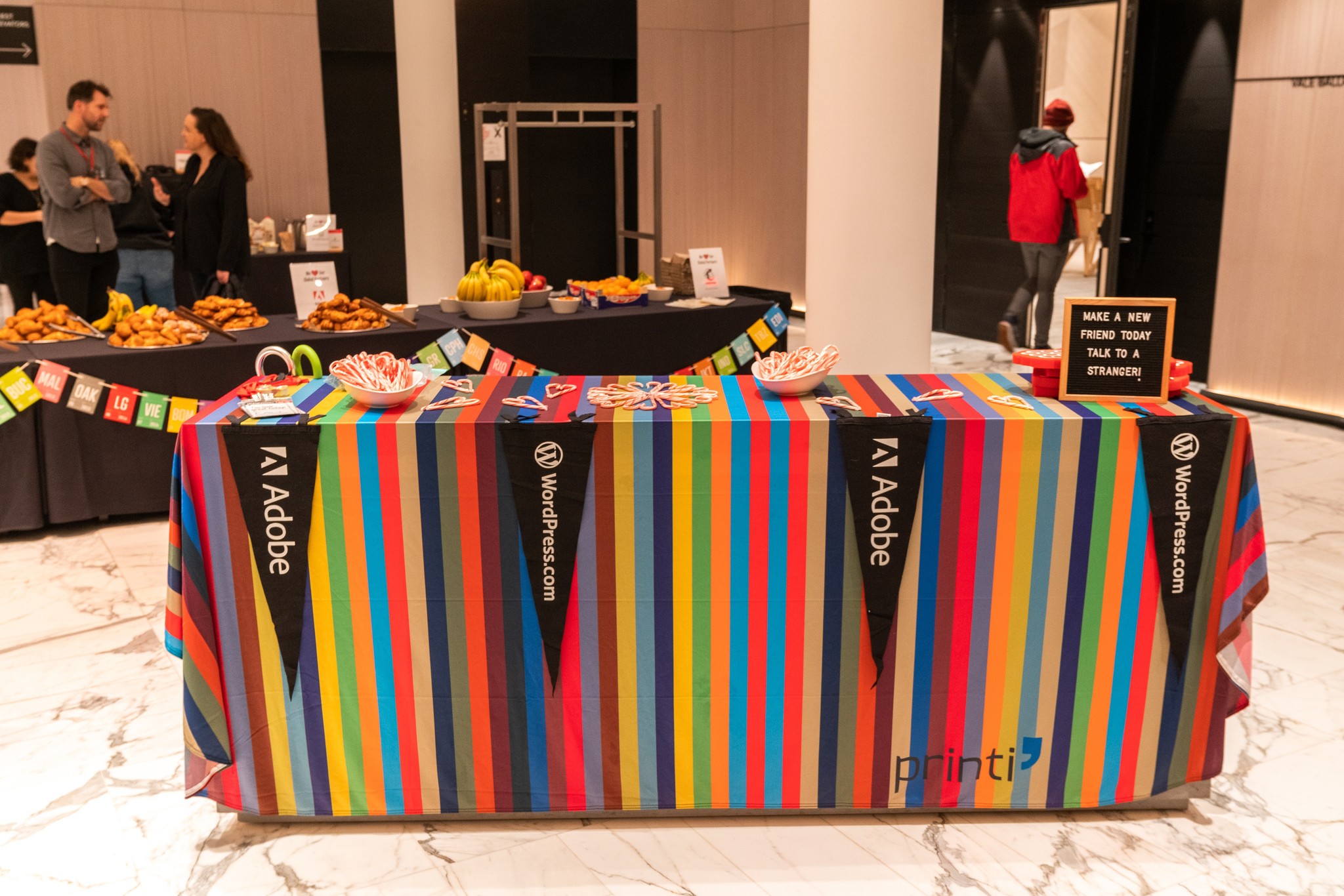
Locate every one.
[0,137,57,315]
[34,80,133,324]
[997,99,1090,355]
[149,108,253,308]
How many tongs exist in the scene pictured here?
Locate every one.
[48,315,106,340]
[360,297,417,328]
[175,304,239,341]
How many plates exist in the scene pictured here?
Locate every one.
[11,336,87,343]
[226,324,263,331]
[309,321,391,332]
[108,330,210,349]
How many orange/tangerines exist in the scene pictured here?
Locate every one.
[573,277,640,297]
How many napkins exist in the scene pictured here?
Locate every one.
[699,296,736,306]
[665,298,709,309]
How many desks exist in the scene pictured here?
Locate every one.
[165,373,1269,823]
[0,245,793,532]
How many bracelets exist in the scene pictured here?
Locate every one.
[81,177,87,187]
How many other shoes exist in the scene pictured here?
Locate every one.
[998,320,1017,353]
[1034,344,1053,350]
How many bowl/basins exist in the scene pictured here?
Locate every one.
[751,360,833,395]
[262,243,279,254]
[340,370,424,408]
[441,296,463,313]
[382,303,419,322]
[519,284,554,308]
[647,286,673,301]
[548,296,582,314]
[251,246,257,254]
[458,297,522,320]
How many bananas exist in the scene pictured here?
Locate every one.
[90,287,157,332]
[632,272,654,286]
[456,256,524,301]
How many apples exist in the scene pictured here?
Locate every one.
[522,270,547,290]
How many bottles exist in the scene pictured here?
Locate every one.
[328,229,344,253]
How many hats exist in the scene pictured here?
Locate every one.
[1042,99,1074,128]
